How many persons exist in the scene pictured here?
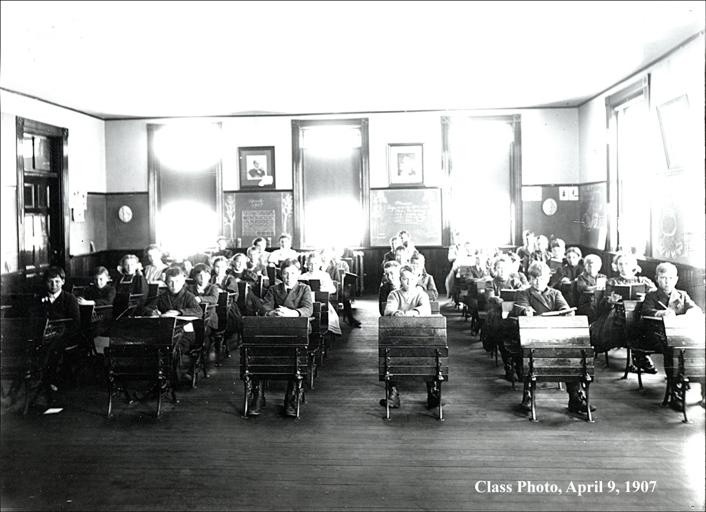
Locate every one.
[78,233,360,381]
[513,260,596,413]
[249,157,266,179]
[32,265,81,410]
[140,266,203,395]
[640,262,706,410]
[380,230,658,374]
[248,258,315,416]
[380,265,440,410]
[399,155,415,176]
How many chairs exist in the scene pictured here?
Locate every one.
[266,267,275,286]
[236,282,248,349]
[239,317,309,420]
[146,284,159,307]
[296,248,706,424]
[249,275,263,317]
[179,303,211,388]
[0,317,53,414]
[111,285,130,318]
[103,318,175,418]
[213,292,228,367]
[79,305,94,345]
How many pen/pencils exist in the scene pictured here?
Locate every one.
[658,301,668,309]
[513,304,537,313]
[155,305,158,313]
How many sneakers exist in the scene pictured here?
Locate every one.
[283,399,296,417]
[379,389,400,408]
[521,392,536,411]
[247,397,265,416]
[568,392,596,412]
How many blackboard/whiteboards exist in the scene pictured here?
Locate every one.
[370,187,445,248]
[222,189,294,251]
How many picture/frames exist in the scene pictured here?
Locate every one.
[388,143,425,188]
[238,146,275,190]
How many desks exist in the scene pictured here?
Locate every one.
[207,304,219,308]
[94,304,113,309]
[229,293,238,297]
[48,318,73,323]
[308,317,315,321]
[128,316,198,321]
[130,293,144,297]
